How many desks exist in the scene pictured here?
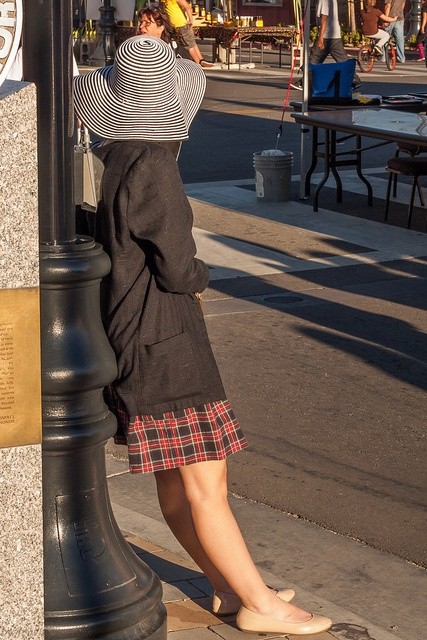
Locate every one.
[116,24,263,64]
[288,107,427,213]
[222,27,297,71]
[290,94,427,201]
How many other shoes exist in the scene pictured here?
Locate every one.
[212,586,295,618]
[374,45,384,54]
[235,602,333,635]
[289,80,303,91]
[199,58,213,67]
[376,58,386,63]
[417,57,426,62]
[352,81,363,90]
[397,59,406,63]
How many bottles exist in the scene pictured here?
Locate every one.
[233,15,263,28]
[201,8,206,16]
[206,11,212,21]
[194,5,200,12]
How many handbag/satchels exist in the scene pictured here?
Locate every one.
[298,58,357,100]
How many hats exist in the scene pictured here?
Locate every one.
[72,36,206,141]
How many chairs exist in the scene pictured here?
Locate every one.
[392,140,427,197]
[384,156,427,229]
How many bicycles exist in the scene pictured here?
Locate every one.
[357,17,397,74]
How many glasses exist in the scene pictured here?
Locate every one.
[138,20,156,26]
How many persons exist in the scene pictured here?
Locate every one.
[74,35,332,635]
[138,6,181,43]
[382,0,406,63]
[359,0,398,57]
[290,0,361,91]
[162,0,203,64]
[416,0,427,61]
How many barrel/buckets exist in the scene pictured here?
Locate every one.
[252,129,295,202]
